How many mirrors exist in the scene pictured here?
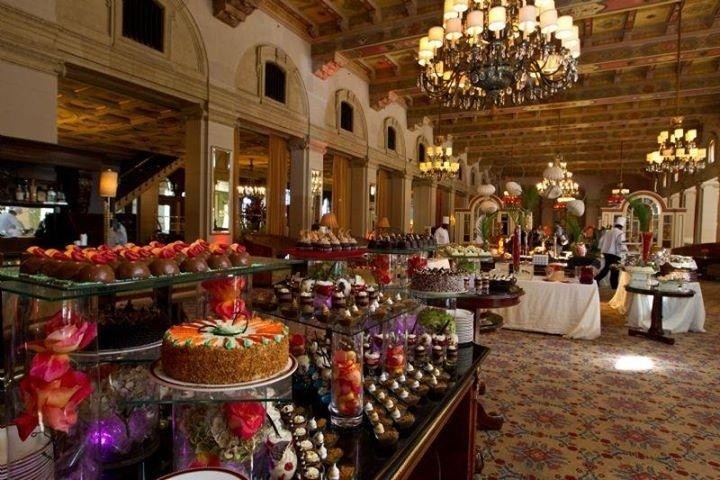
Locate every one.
[310,168,323,231]
[207,144,233,236]
[367,182,378,232]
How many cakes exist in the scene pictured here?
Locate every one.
[161,311,290,385]
[367,231,435,249]
[295,227,358,252]
[446,245,491,257]
[84,300,188,350]
[479,269,513,281]
[18,236,253,283]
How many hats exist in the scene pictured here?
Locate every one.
[615,217,625,227]
[443,217,450,224]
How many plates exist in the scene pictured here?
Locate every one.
[456,346,473,375]
[146,350,299,392]
[157,469,248,480]
[0,421,58,480]
[447,308,475,345]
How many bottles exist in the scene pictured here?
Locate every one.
[384,312,408,379]
[110,221,126,245]
[332,434,359,480]
[332,329,367,428]
[14,177,66,203]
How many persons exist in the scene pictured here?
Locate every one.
[434,216,450,245]
[594,224,628,290]
[513,221,604,268]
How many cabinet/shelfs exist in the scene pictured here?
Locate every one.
[1,229,526,479]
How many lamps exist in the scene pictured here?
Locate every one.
[534,107,581,197]
[419,99,461,185]
[376,216,392,231]
[413,0,582,111]
[645,1,708,180]
[99,168,119,242]
[319,213,338,230]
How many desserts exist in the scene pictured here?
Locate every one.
[274,267,471,480]
